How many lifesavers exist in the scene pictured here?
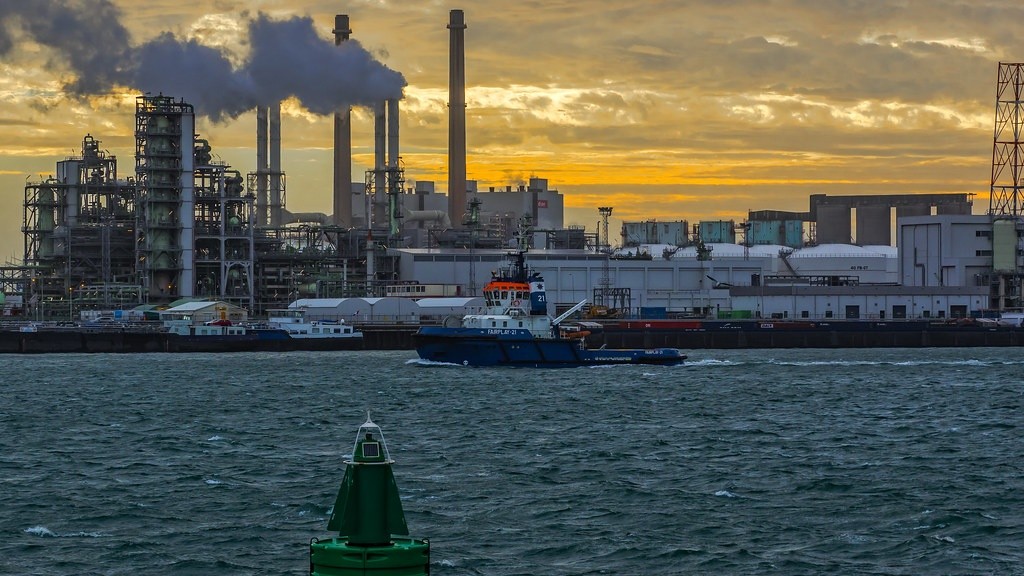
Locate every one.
[562,331,590,338]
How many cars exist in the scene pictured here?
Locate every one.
[204,319,217,326]
[207,319,232,326]
[94,317,114,323]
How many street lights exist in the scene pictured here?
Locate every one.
[977,276,983,318]
[569,273,574,302]
[70,288,72,321]
[212,272,218,319]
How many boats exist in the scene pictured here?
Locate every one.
[167,310,364,351]
[410,246,687,368]
